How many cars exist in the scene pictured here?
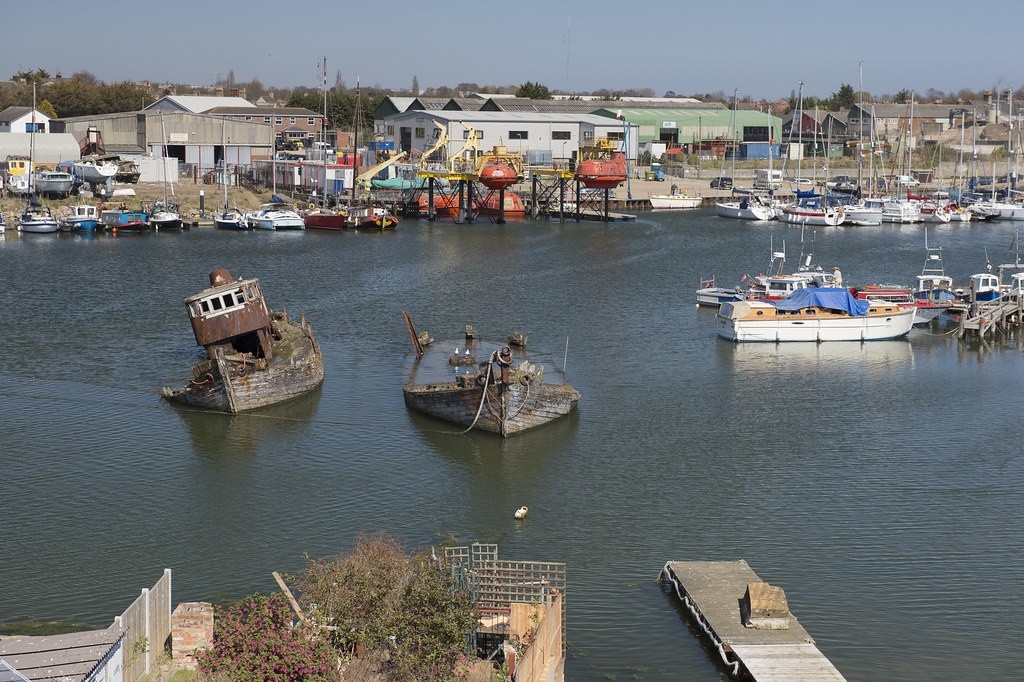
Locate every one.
[574,186,616,198]
[710,177,732,190]
[794,179,811,185]
[866,176,890,193]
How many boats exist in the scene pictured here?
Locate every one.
[695,221,842,309]
[715,276,918,343]
[157,269,326,415]
[0,54,539,235]
[403,311,582,439]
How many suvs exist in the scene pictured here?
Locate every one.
[894,175,921,187]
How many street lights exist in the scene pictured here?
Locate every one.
[19,78,37,209]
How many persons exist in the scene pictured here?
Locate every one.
[616,108,622,120]
[343,152,348,164]
[833,267,842,283]
[807,275,823,288]
[671,184,678,195]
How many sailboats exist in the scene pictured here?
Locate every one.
[693,61,1024,229]
[648,132,704,210]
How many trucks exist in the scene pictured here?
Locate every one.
[752,170,783,190]
